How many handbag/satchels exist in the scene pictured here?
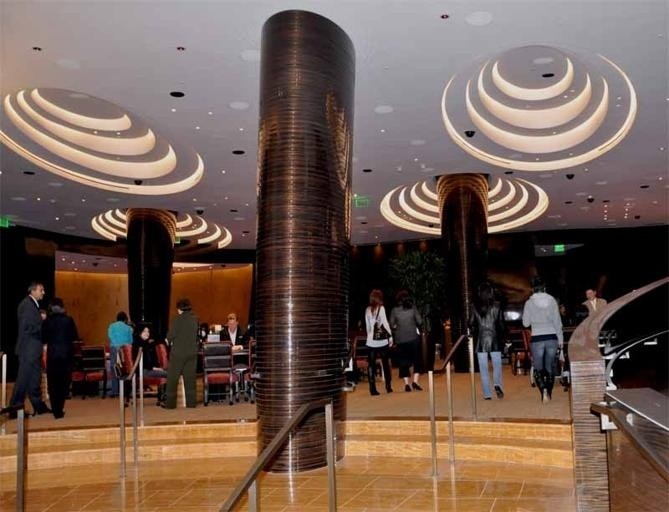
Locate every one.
[373,322,391,340]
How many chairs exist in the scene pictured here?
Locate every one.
[506,328,532,375]
[351,330,369,369]
[64,337,258,408]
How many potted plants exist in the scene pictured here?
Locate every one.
[390,245,453,374]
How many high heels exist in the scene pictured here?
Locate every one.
[412,382,422,391]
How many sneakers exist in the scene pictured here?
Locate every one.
[495,386,503,398]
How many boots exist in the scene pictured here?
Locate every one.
[368,366,380,395]
[533,367,554,404]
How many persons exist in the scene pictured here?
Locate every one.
[583,286,608,315]
[469,285,507,400]
[11,283,46,420]
[365,290,394,394]
[108,299,253,410]
[40,297,77,418]
[523,277,565,404]
[390,290,425,393]
